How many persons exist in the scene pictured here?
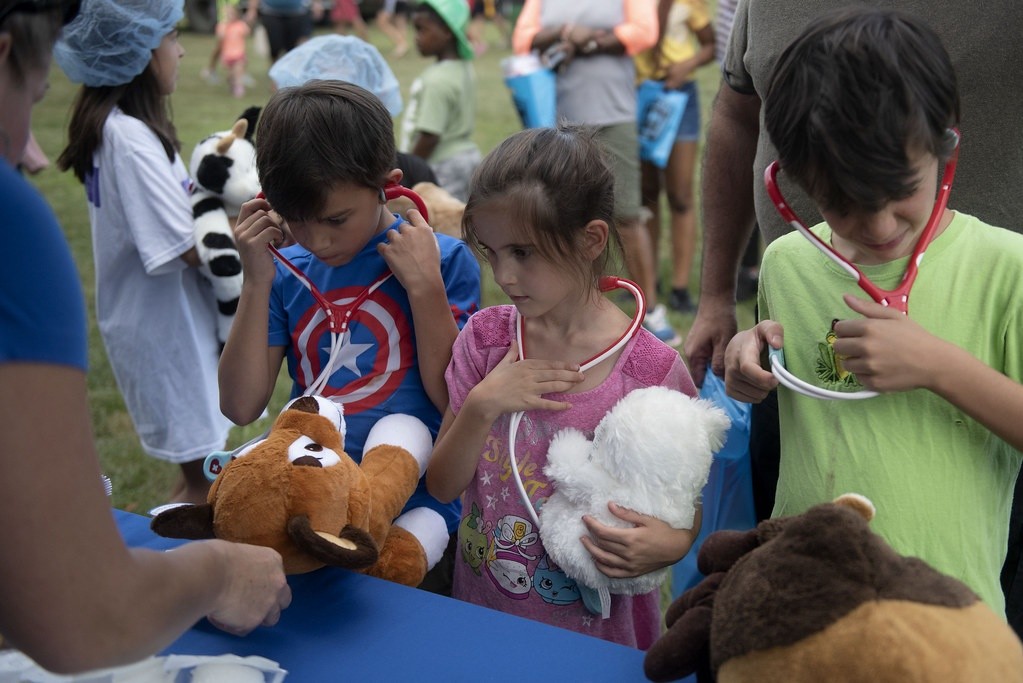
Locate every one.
[0,0,1023,671]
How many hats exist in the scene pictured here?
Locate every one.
[417,0,478,60]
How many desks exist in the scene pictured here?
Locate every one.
[109,504,700,683]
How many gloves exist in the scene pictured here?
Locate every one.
[528,22,626,71]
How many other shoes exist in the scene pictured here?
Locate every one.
[641,301,683,348]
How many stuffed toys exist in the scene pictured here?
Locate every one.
[186,106,262,343]
[389,183,468,239]
[644,493,1023,682]
[540,385,731,596]
[151,394,450,588]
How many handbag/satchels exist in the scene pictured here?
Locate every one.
[503,48,560,130]
[636,77,689,170]
